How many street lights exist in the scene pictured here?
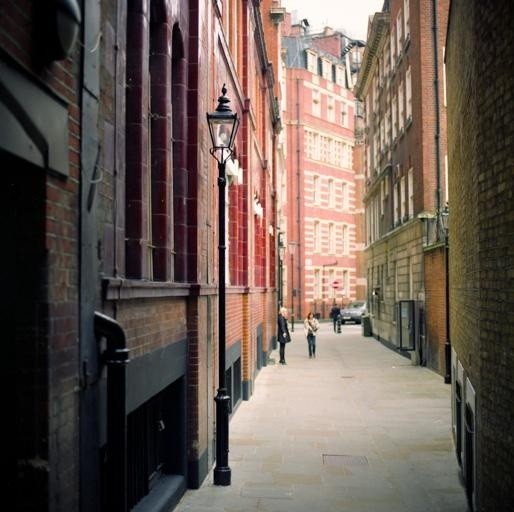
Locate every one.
[202,80,243,490]
[288,238,300,334]
[438,198,452,386]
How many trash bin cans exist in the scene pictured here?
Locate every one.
[315,313,320,319]
[362,316,370,336]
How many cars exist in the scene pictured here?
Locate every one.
[339,300,368,326]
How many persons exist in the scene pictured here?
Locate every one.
[331,303,341,333]
[304,312,319,358]
[277,307,291,366]
